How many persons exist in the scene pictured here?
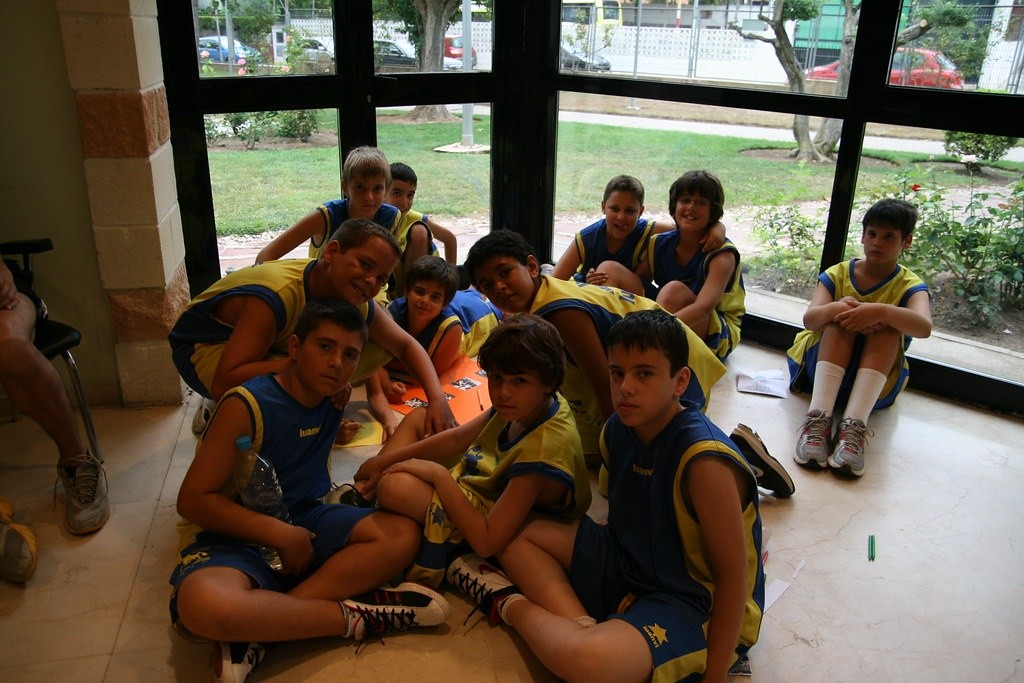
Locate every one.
[786,197,934,479]
[463,228,796,497]
[0,252,111,582]
[383,162,470,301]
[446,307,765,683]
[253,147,403,303]
[364,254,556,398]
[354,313,593,588]
[557,172,726,287]
[596,170,746,364]
[165,294,451,683]
[192,370,400,445]
[169,217,461,440]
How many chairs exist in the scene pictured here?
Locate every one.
[0,238,104,466]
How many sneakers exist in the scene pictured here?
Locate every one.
[791,408,834,470]
[0,495,39,583]
[826,416,875,480]
[341,581,451,656]
[52,449,112,535]
[210,639,268,683]
[726,422,796,503]
[191,397,216,434]
[445,552,524,626]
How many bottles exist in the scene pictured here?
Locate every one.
[235,436,294,570]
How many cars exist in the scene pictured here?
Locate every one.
[561,38,610,71]
[783,47,966,90]
[443,33,478,68]
[198,35,261,65]
[372,37,464,70]
[297,35,334,63]
